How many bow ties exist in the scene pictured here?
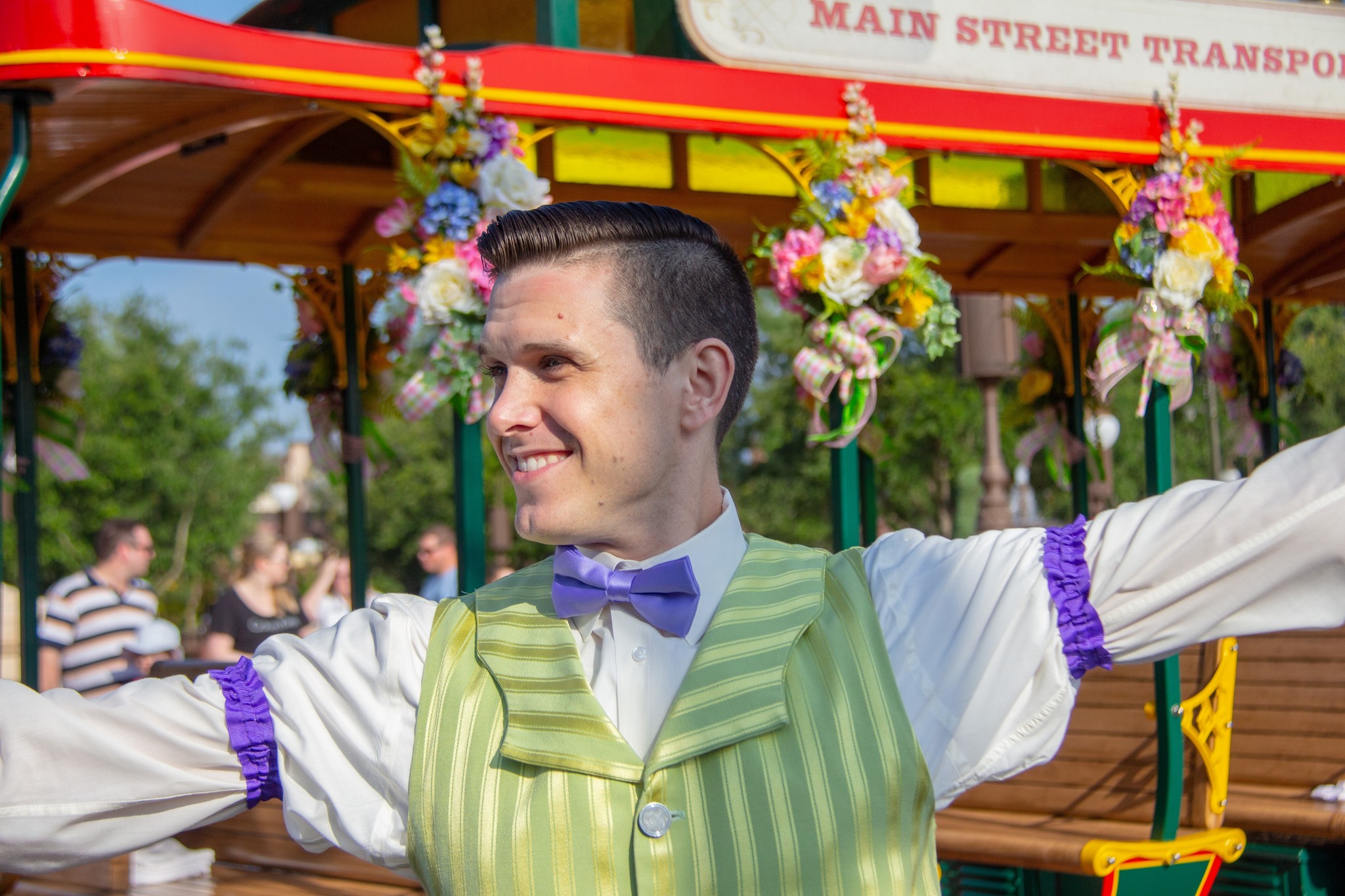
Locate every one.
[552,543,700,640]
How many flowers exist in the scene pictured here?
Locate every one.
[741,79,963,457]
[999,65,1306,480]
[280,21,553,474]
[0,250,85,427]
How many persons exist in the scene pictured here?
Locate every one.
[35,520,185,700]
[298,547,384,638]
[202,531,314,666]
[413,524,462,604]
[0,201,1345,896]
[487,562,517,587]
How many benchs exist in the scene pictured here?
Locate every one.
[0,598,1345,896]
